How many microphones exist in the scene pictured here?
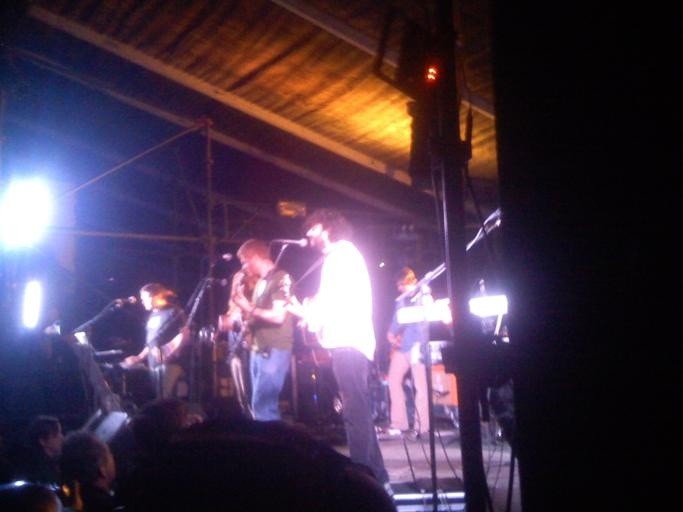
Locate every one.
[275,238,308,248]
[116,296,137,304]
[211,253,232,262]
[205,278,228,287]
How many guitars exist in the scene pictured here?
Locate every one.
[227,274,254,347]
[390,282,433,357]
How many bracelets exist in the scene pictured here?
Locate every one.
[249,307,256,314]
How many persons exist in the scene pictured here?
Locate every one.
[385,264,434,442]
[121,277,198,403]
[282,205,397,503]
[229,238,297,426]
[206,268,254,419]
[1,394,397,512]
